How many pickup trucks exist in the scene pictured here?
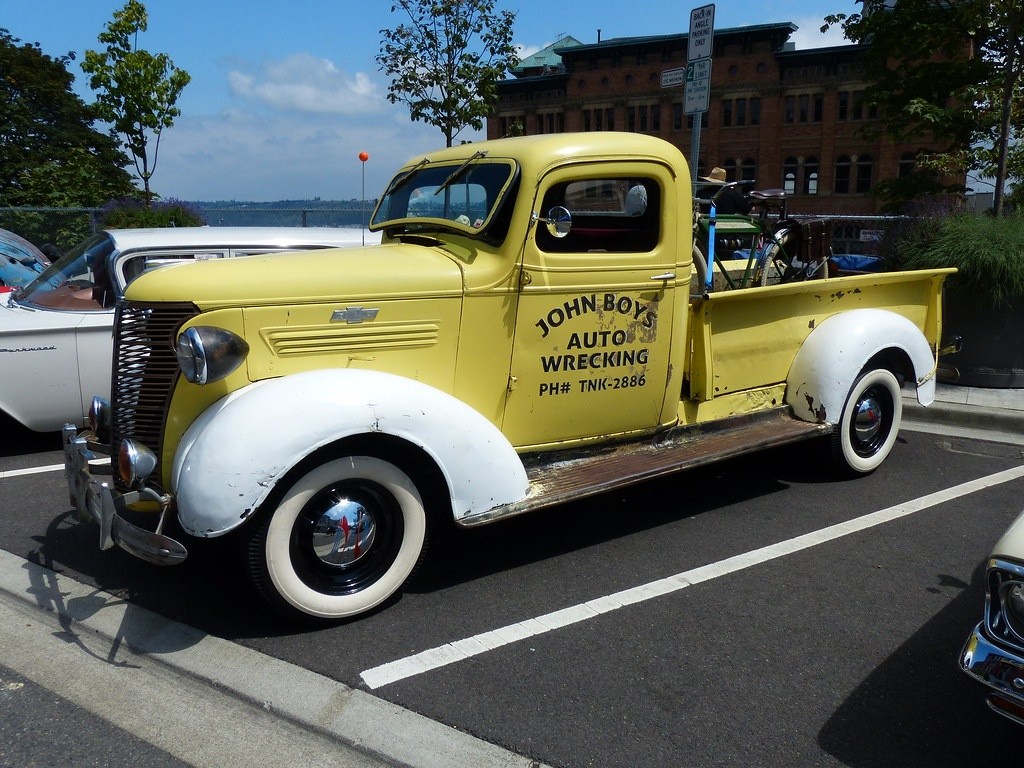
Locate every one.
[62,133,965,627]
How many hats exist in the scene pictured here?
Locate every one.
[701,167,728,183]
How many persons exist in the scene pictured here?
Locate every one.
[624,185,647,216]
[696,167,752,214]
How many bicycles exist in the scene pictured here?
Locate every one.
[686,178,836,296]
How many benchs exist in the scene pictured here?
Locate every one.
[537,226,659,254]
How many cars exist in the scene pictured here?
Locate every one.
[0,226,385,449]
[0,226,68,290]
[960,506,1024,728]
